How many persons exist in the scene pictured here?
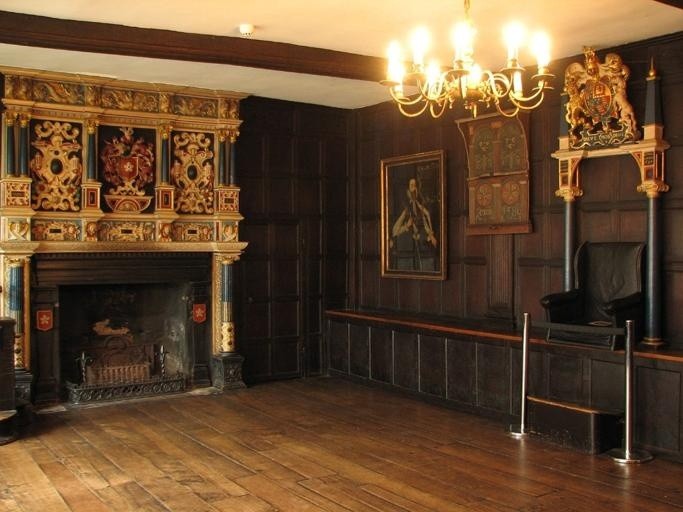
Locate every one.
[391,178,437,272]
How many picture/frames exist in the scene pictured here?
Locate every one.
[379,148,448,281]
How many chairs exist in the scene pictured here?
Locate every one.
[540,240,647,350]
